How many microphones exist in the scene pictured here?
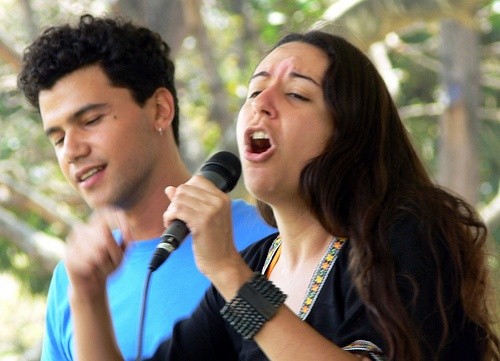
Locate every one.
[149,151,240,272]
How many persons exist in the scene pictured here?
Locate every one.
[63,31,500,361]
[17,14,278,361]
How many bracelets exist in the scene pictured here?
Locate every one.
[218,273,287,339]
